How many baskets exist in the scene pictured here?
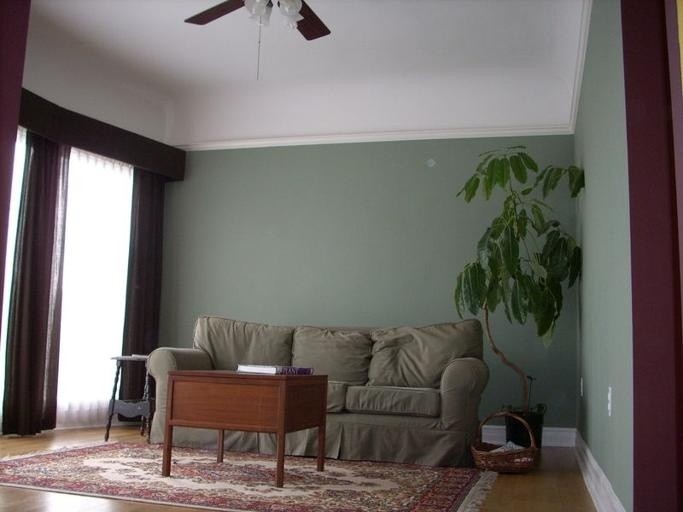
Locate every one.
[471,439,540,475]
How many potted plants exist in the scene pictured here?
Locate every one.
[452,144,585,450]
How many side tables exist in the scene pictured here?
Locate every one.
[104,354,152,443]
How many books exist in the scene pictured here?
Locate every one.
[237,364,314,374]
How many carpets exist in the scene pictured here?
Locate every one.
[0,440,500,512]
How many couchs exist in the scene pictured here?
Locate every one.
[145,314,489,470]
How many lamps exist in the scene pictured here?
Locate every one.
[241,1,305,32]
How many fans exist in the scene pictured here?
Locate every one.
[183,1,331,42]
